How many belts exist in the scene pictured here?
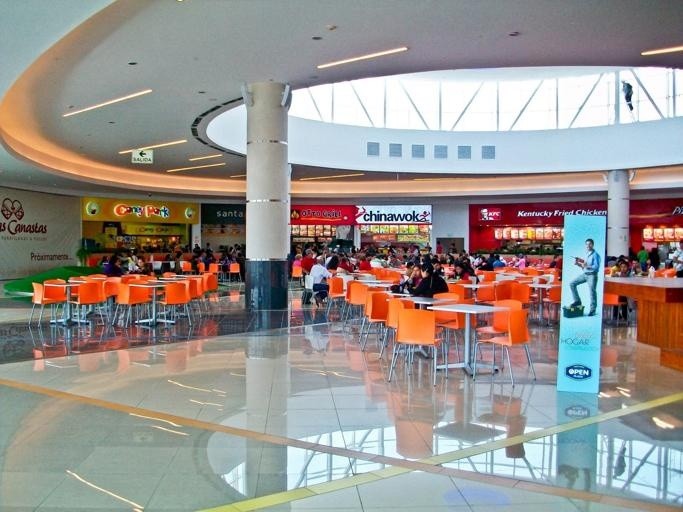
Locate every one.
[585,272,597,275]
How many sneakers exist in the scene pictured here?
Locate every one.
[571,301,583,308]
[588,309,597,316]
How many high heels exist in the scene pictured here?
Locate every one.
[314,294,326,307]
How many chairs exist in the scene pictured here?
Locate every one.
[25,252,242,334]
[290,256,562,386]
[27,318,222,379]
[603,267,676,328]
[360,385,530,464]
